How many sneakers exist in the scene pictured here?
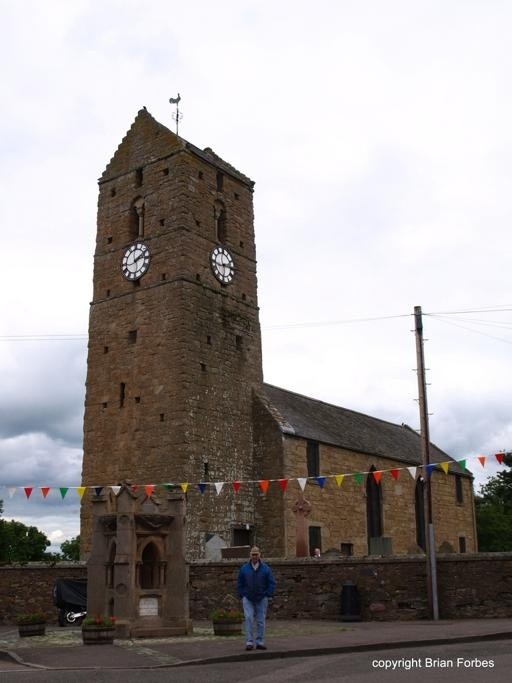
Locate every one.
[247,645,252,650]
[256,646,266,650]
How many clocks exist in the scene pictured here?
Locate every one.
[120,241,152,282]
[210,245,236,285]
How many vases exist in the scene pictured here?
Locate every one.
[82,624,116,645]
[213,617,242,636]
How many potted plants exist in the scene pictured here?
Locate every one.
[17,608,47,637]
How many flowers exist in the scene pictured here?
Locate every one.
[82,615,117,625]
[208,607,244,619]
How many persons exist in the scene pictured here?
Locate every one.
[237,545,275,651]
[314,548,322,557]
